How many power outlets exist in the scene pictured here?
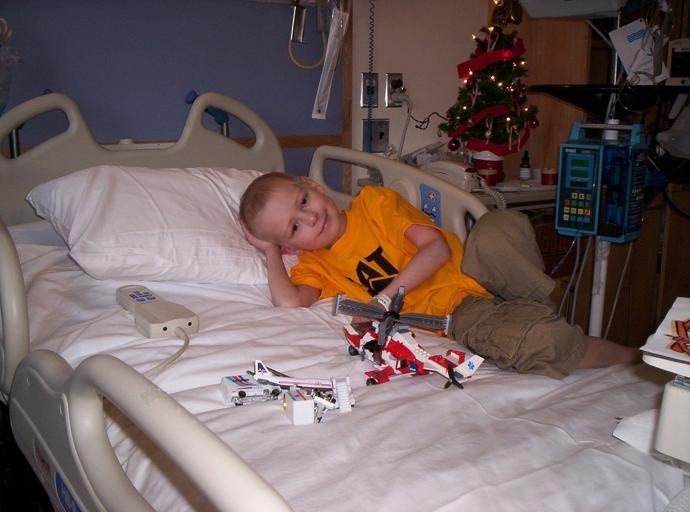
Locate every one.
[385,73,403,108]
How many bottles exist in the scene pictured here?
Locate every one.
[520,148,532,180]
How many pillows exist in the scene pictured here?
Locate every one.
[25,164,299,286]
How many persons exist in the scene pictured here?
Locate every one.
[239,172,642,381]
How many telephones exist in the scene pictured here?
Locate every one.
[420,159,484,199]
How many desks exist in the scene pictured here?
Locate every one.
[449,172,673,345]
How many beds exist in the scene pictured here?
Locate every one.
[0,93,689,511]
